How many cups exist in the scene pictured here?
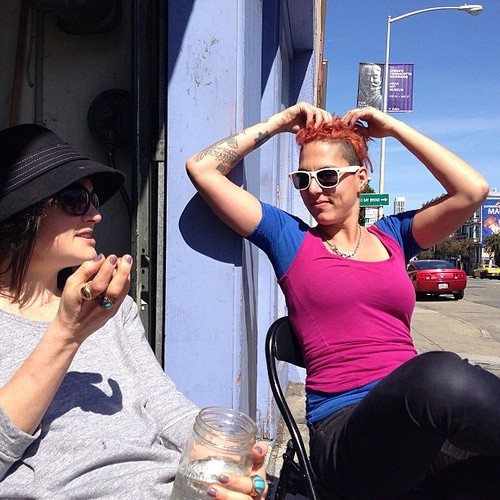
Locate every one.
[168,406,258,500]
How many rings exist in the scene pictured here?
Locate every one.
[99,295,117,309]
[78,280,97,301]
[248,473,266,500]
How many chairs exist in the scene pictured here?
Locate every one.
[264,316,319,499]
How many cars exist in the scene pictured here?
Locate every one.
[406,260,467,301]
[472,264,500,280]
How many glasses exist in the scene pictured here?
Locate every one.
[288,166,360,191]
[52,182,99,217]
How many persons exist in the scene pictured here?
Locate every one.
[184,101,500,500]
[0,123,269,500]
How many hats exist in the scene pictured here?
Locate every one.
[0,124,126,220]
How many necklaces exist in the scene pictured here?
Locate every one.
[318,222,362,259]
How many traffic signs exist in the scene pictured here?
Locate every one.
[360,193,390,207]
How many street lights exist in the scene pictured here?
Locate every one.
[378,3,484,220]
